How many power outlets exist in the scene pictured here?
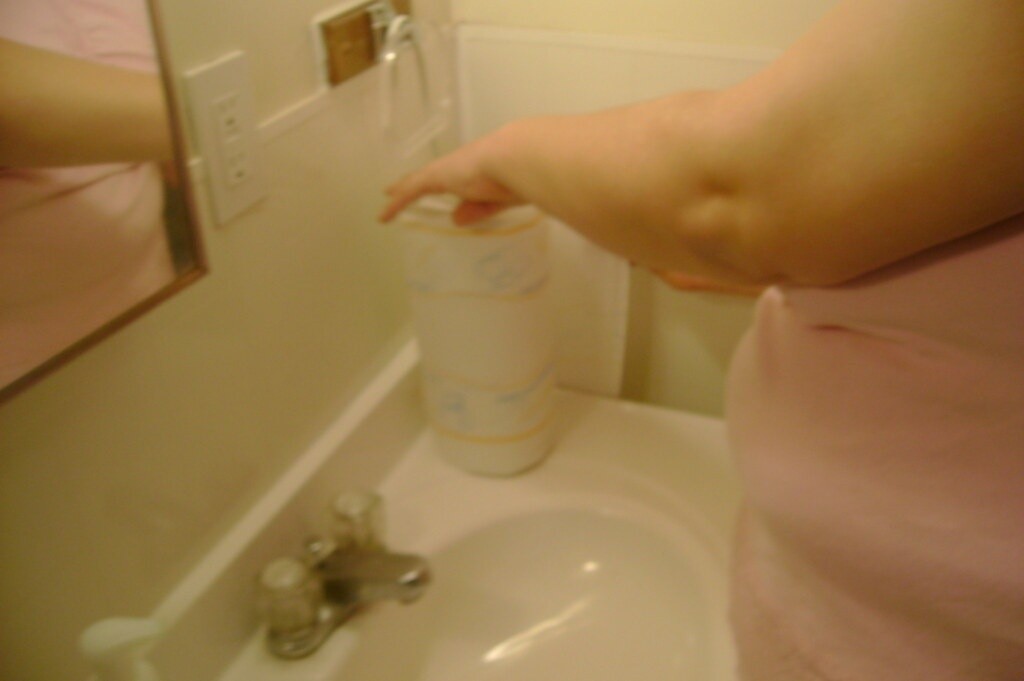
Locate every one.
[185,49,265,227]
[319,0,413,87]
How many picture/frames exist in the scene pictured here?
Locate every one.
[0,0,212,405]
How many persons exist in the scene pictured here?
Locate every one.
[374,0,1024,681]
[0,0,178,392]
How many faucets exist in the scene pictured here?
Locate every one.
[305,527,432,609]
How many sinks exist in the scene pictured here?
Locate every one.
[297,483,736,680]
